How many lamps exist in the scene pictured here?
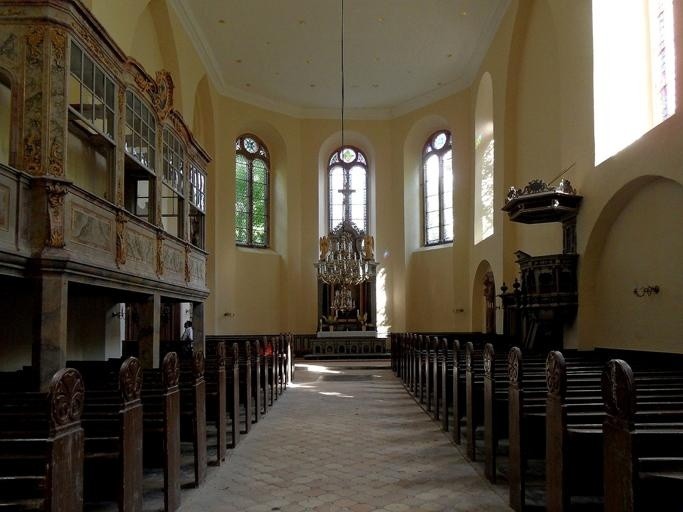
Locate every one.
[310,1,376,309]
